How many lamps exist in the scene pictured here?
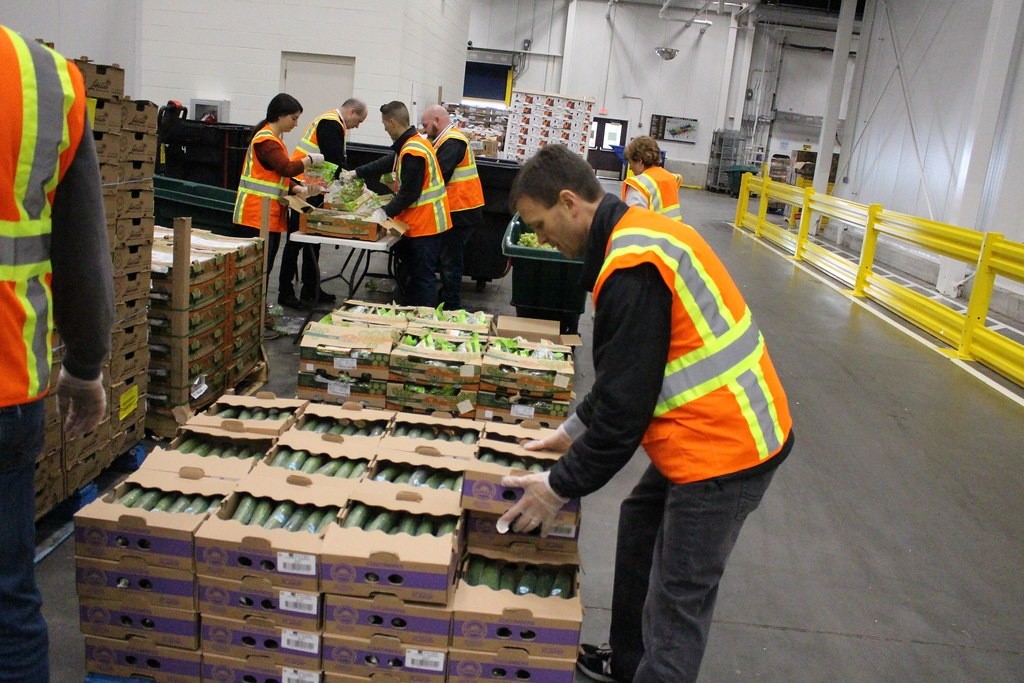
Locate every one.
[654,20,679,62]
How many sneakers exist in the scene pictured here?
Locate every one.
[577,642,616,683]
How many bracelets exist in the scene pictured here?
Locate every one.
[307,154,313,165]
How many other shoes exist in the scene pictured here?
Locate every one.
[278,294,302,307]
[300,288,336,302]
[264,327,280,339]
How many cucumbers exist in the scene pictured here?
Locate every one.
[107,405,575,599]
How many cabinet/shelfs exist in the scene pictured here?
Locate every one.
[705,129,748,197]
[442,101,508,136]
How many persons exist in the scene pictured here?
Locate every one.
[277,97,368,310]
[495,143,796,683]
[0,21,117,682]
[232,92,325,341]
[420,103,486,310]
[619,135,685,225]
[339,100,455,309]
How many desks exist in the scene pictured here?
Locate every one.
[289,227,404,346]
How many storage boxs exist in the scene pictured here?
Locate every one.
[282,195,388,242]
[73,300,584,683]
[478,139,498,156]
[31,37,267,526]
